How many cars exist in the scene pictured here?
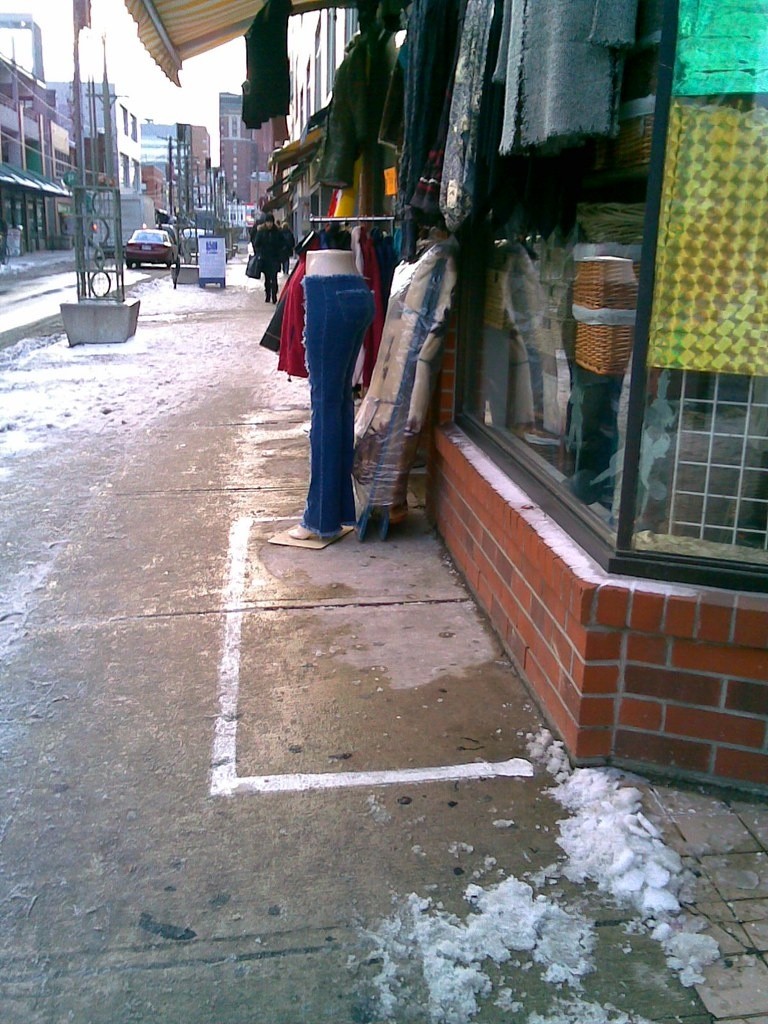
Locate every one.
[155,208,213,255]
[126,229,178,269]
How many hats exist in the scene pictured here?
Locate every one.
[264,214,275,223]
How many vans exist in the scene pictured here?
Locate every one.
[92,193,156,259]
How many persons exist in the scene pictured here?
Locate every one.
[288,250,378,540]
[249,214,293,304]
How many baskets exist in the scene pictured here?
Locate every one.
[569,318,633,378]
[566,242,640,313]
[609,96,659,167]
[575,198,645,244]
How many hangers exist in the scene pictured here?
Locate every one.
[309,214,390,239]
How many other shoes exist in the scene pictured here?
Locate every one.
[265,294,270,302]
[283,271,285,274]
[286,271,288,275]
[271,294,278,304]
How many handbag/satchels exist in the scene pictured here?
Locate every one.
[245,252,262,280]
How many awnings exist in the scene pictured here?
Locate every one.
[123,0,353,85]
[267,100,332,171]
[262,167,307,213]
[0,163,72,198]
